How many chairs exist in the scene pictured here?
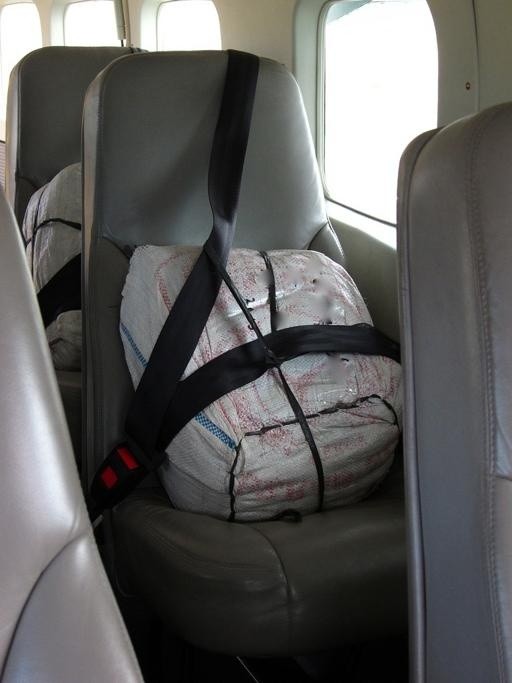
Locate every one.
[0,193,146,681]
[80,47,423,681]
[3,41,154,223]
[396,100,512,682]
